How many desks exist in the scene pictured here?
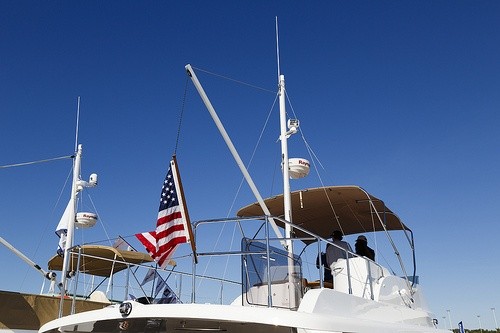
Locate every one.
[332,256,384,299]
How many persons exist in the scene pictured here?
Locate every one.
[326,231,354,269]
[316,243,333,283]
[355,236,375,262]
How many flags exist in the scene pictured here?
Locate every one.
[135,165,188,270]
